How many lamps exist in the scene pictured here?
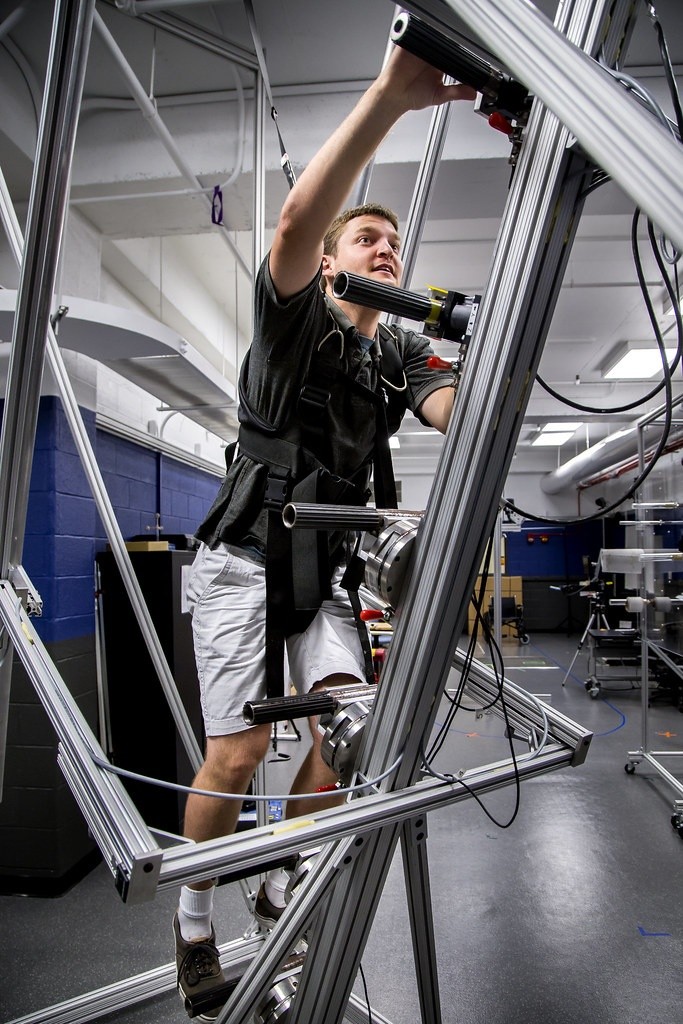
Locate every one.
[663,284,683,316]
[531,422,583,446]
[601,339,679,379]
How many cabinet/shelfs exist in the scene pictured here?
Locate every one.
[98,549,209,836]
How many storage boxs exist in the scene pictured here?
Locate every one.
[125,541,168,551]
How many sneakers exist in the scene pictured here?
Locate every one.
[171,908,228,1023]
[253,884,287,931]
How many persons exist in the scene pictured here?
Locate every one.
[170,8,477,1024]
